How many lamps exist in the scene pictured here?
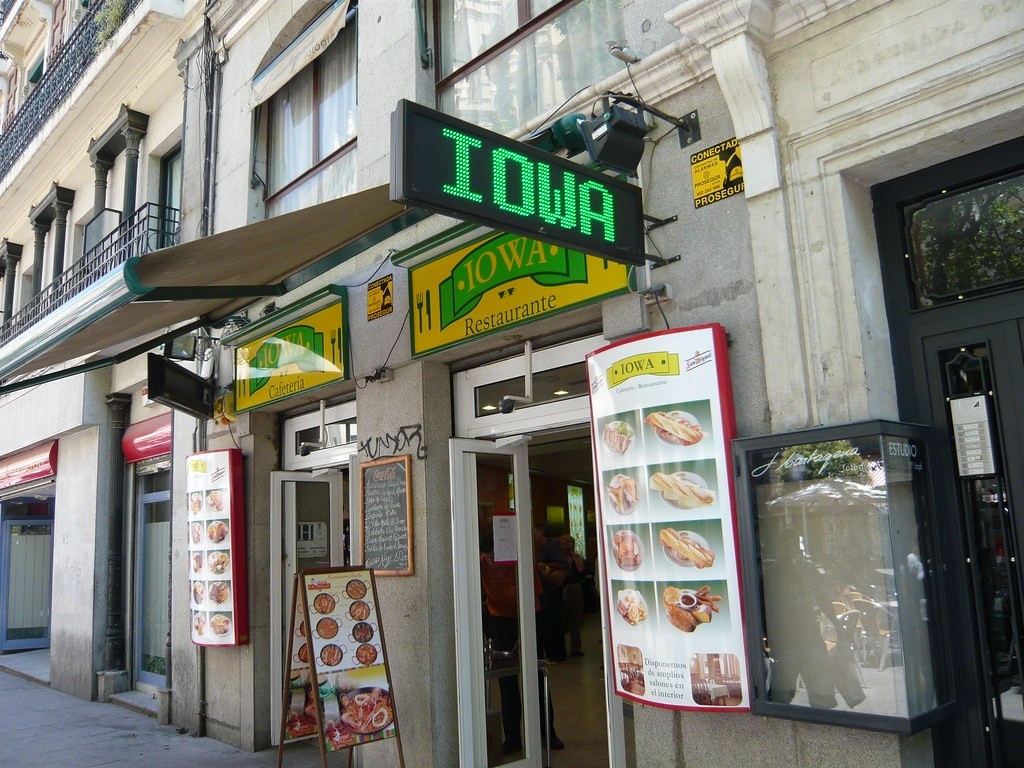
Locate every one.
[575,89,649,179]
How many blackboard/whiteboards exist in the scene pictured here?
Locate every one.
[358,454,415,576]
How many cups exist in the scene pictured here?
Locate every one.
[675,593,699,613]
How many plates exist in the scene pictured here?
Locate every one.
[194,612,206,636]
[663,530,712,570]
[193,552,205,574]
[612,529,645,573]
[603,420,635,454]
[608,474,640,515]
[660,472,708,510]
[193,582,205,607]
[191,522,204,544]
[654,410,701,449]
[191,493,203,516]
[208,551,228,575]
[209,581,229,605]
[617,589,648,624]
[666,589,709,616]
[207,521,228,545]
[211,614,232,638]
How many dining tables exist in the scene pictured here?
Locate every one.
[636,670,644,682]
[620,671,629,685]
[692,682,728,704]
[721,680,742,697]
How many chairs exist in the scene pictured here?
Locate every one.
[814,581,893,708]
[619,661,644,693]
[692,671,742,705]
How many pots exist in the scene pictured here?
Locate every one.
[352,643,382,666]
[292,642,309,664]
[341,578,370,600]
[295,603,306,637]
[309,593,340,614]
[337,687,394,735]
[348,621,378,643]
[312,617,343,639]
[344,600,374,622]
[316,644,348,666]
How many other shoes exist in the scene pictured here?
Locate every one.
[550,733,564,750]
[571,651,584,657]
[502,740,522,755]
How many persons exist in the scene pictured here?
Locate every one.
[479,517,588,753]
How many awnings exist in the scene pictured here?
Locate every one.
[0,114,585,394]
